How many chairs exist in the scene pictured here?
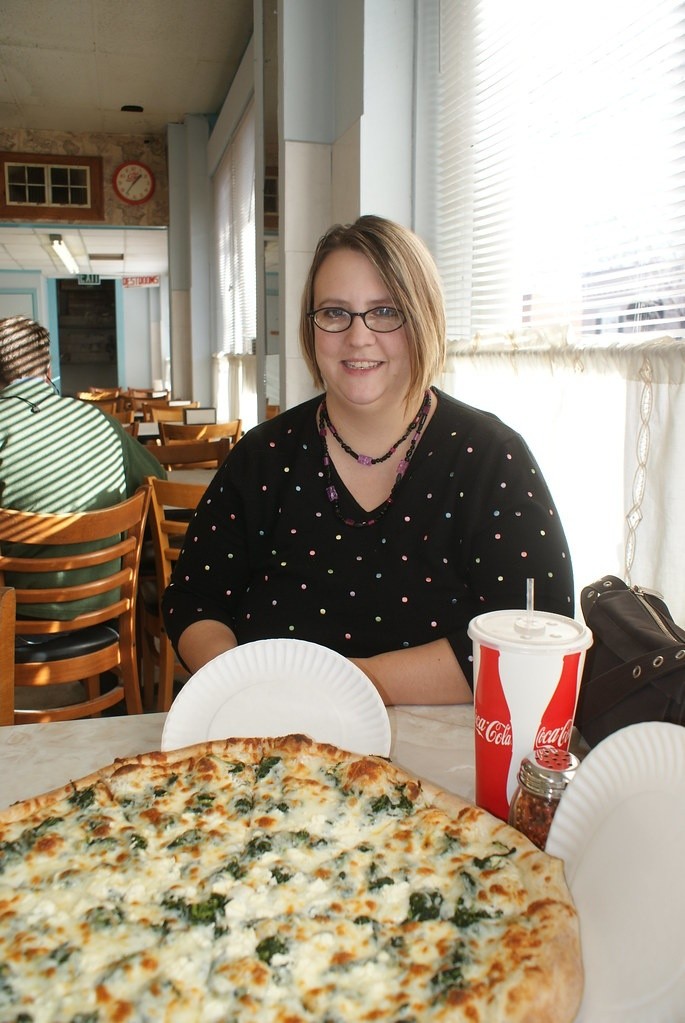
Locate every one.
[158,418,242,472]
[76,388,170,414]
[110,411,135,423]
[124,422,139,438]
[140,437,231,470]
[149,401,200,423]
[136,475,209,714]
[0,484,152,728]
[142,403,167,422]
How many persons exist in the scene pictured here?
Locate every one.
[160,214,573,707]
[0,315,168,657]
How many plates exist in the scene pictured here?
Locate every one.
[541,721,685,1023]
[160,638,391,759]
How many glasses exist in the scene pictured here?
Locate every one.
[306,306,405,334]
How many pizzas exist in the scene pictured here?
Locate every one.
[0,734,584,1023]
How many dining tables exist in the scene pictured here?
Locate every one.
[166,470,218,485]
[134,411,144,417]
[0,706,475,809]
[168,400,190,407]
[121,422,185,438]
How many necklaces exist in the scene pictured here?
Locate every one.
[318,390,432,527]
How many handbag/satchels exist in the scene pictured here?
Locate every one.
[571,574,685,751]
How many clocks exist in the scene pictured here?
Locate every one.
[113,162,156,205]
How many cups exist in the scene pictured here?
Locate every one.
[468,609,594,825]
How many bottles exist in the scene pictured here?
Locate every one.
[508,747,579,849]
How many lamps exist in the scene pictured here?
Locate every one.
[49,234,80,275]
